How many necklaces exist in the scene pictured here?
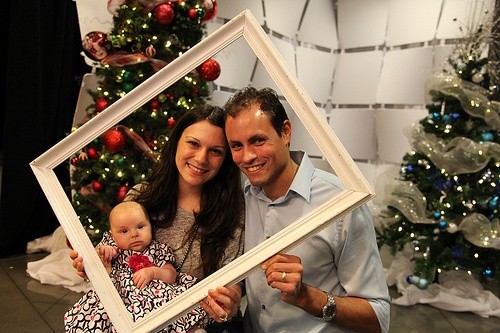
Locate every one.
[173,224,199,271]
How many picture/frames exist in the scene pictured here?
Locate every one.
[29,8,376,333]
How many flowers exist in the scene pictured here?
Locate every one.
[128,252,153,273]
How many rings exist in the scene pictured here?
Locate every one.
[220,313,229,321]
[281,272,287,284]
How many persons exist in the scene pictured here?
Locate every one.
[70,104,247,333]
[63,202,210,333]
[226,88,391,333]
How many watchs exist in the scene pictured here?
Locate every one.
[315,291,337,322]
